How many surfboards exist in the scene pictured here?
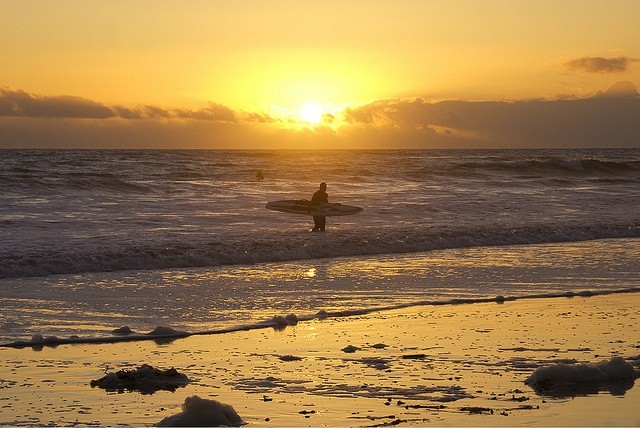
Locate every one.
[265,199,364,215]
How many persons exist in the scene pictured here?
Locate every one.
[310,182,330,231]
